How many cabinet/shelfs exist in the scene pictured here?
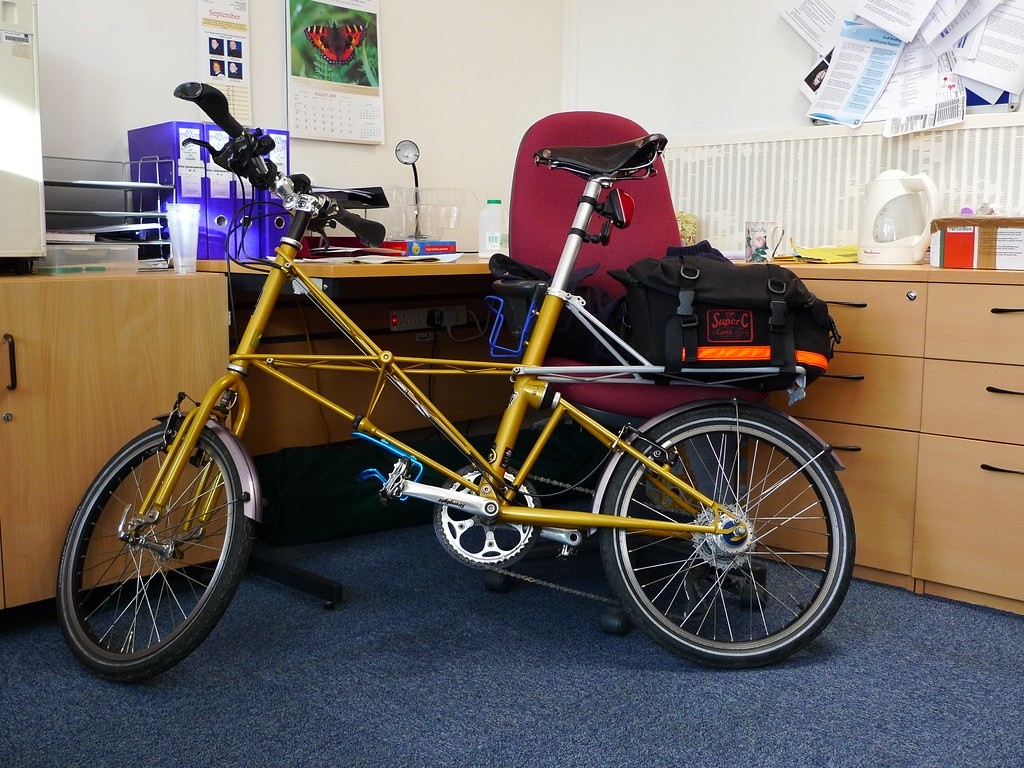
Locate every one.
[744,261,1024,619]
[0,272,230,610]
[43,156,176,269]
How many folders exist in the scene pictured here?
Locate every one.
[128,121,291,262]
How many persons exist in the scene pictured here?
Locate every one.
[209,38,243,79]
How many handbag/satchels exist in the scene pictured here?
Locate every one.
[598,254,840,389]
[488,253,612,366]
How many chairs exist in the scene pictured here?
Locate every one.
[480,111,770,612]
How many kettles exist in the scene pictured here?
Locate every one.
[857,170,942,264]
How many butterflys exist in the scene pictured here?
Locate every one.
[304,19,368,66]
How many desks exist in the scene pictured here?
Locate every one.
[197,255,518,457]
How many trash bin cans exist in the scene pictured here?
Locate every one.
[644,433,746,519]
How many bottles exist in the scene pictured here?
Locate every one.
[477,199,509,259]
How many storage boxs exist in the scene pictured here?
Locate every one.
[128,122,209,260]
[235,129,262,260]
[205,124,237,260]
[261,129,291,258]
[32,244,139,275]
[930,217,1024,271]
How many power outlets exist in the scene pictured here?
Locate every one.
[390,305,467,331]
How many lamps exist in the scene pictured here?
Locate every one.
[395,140,430,239]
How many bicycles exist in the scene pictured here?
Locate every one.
[52,78,862,679]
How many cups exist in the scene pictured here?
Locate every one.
[372,187,461,240]
[744,221,785,263]
[165,204,201,275]
